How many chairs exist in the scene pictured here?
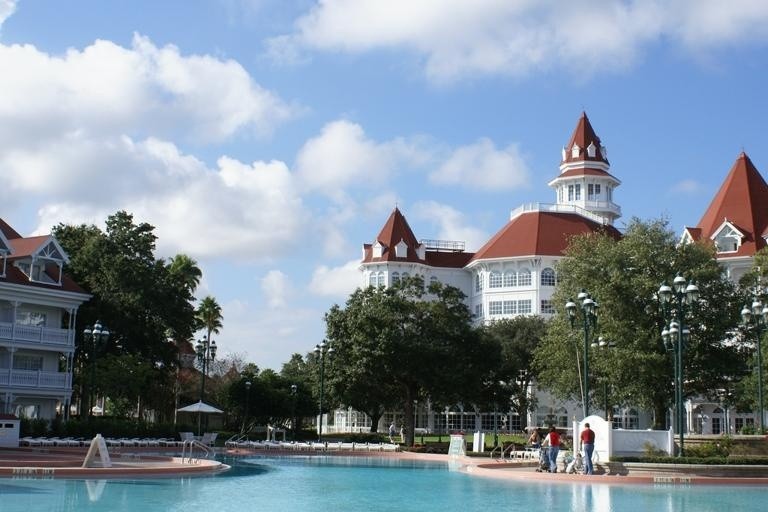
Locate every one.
[223,435,402,453]
[18,433,219,451]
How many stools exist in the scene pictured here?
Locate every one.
[509,450,540,459]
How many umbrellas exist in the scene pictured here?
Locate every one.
[177,400,224,441]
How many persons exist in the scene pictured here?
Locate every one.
[388,422,406,443]
[527,423,595,475]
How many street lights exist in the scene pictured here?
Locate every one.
[194,334,217,435]
[314,339,335,442]
[658,267,699,457]
[566,288,617,421]
[83,318,110,415]
[741,297,768,435]
[245,381,251,441]
[291,385,298,440]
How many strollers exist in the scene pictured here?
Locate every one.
[536,447,551,473]
[560,450,586,475]
